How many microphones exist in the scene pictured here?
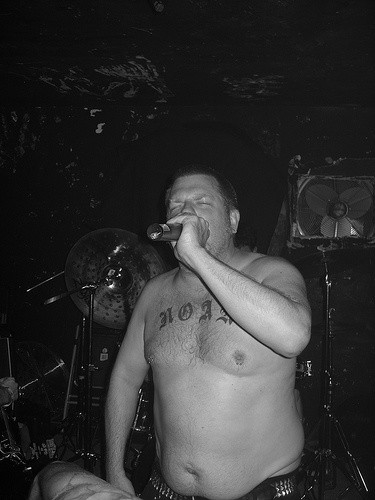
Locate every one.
[148,224,182,242]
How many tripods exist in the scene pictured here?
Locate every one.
[285,156,375,500]
[59,315,104,462]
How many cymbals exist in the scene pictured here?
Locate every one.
[311,321,362,336]
[292,247,373,279]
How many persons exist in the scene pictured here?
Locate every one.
[102,168,316,500]
[0,305,142,500]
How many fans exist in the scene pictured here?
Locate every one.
[288,174,375,252]
